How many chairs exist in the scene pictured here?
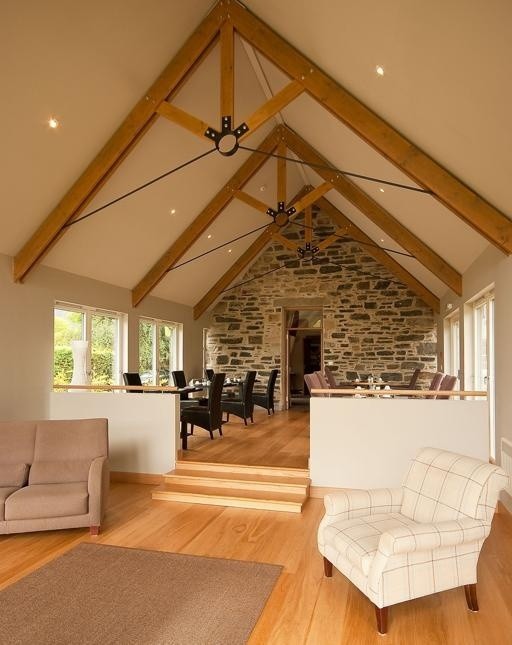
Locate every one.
[304,367,458,399]
[123,369,278,449]
[317,447,508,636]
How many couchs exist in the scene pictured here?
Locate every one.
[0,418,109,535]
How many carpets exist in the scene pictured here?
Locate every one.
[0,542,284,645]
